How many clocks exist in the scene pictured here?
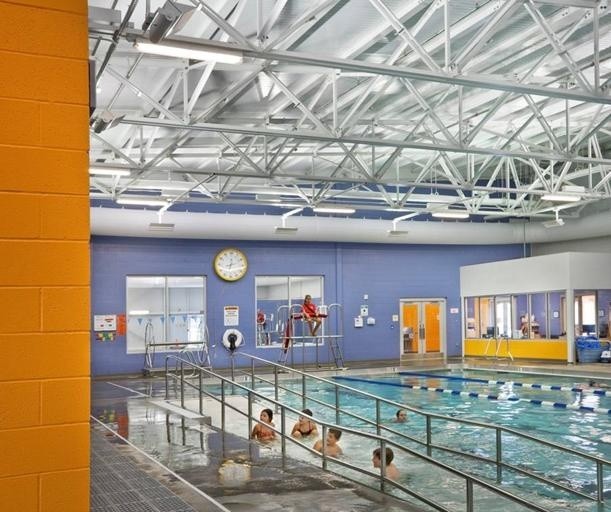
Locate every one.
[213,248,248,282]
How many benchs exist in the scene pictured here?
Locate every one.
[148,398,204,447]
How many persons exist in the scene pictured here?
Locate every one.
[257,305,270,338]
[248,393,407,485]
[299,293,321,338]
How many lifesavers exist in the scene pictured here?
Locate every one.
[223,329,243,348]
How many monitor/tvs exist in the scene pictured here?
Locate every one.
[582,325,595,335]
[487,327,499,335]
